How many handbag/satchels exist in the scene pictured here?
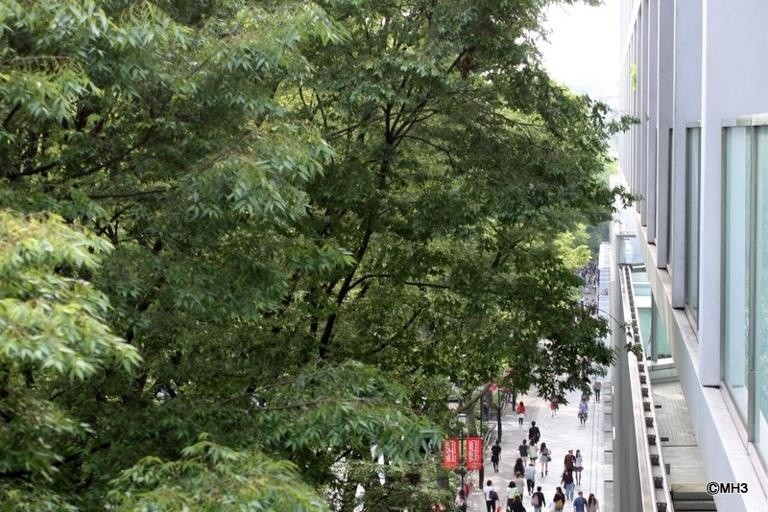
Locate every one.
[489,491,498,500]
[547,455,551,462]
[531,493,538,506]
[555,499,563,510]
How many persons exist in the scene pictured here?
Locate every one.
[454,258,601,512]
